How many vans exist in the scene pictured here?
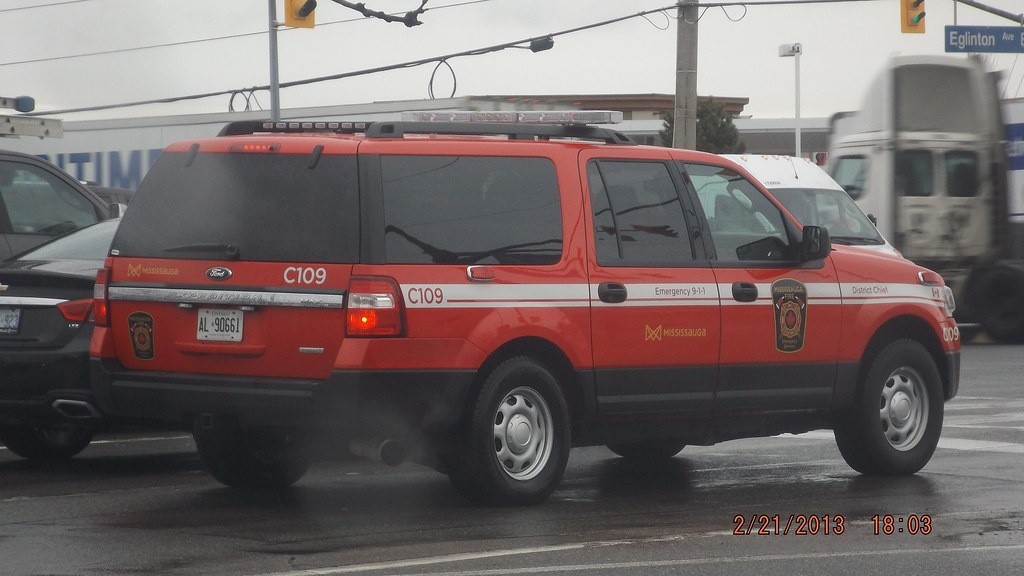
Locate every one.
[685,153,906,258]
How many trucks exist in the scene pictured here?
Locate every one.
[816,53,1024,350]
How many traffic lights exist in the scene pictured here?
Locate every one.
[283,0,318,29]
[899,0,927,34]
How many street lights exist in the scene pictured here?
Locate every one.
[777,41,804,158]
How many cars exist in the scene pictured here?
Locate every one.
[0,208,189,466]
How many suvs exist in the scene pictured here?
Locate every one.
[89,107,964,509]
[0,149,137,264]
